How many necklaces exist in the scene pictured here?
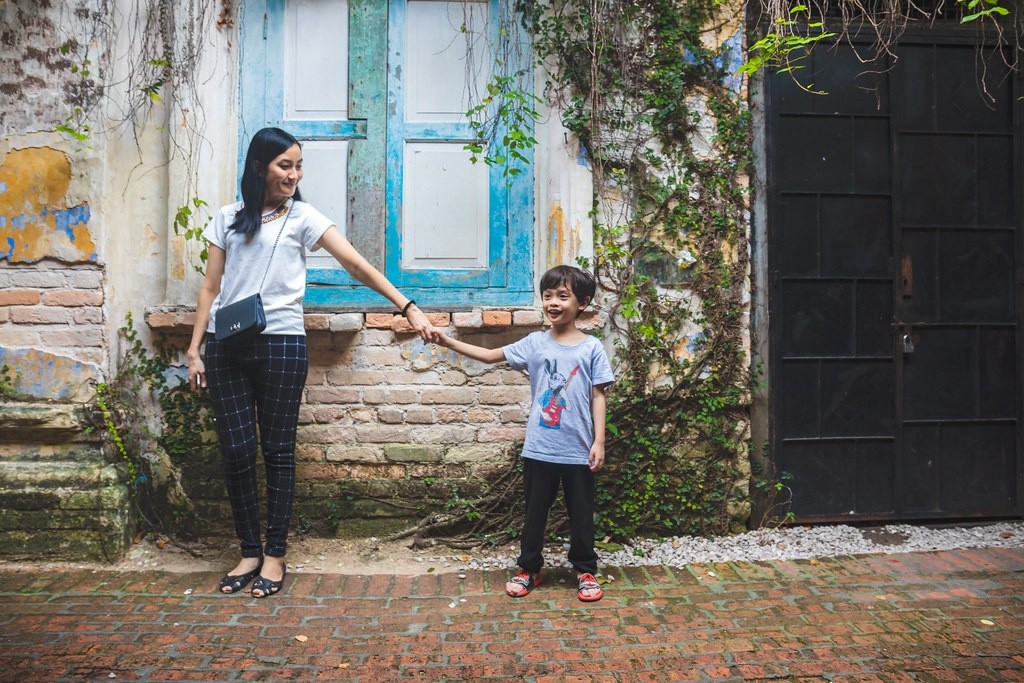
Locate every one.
[234,206,289,224]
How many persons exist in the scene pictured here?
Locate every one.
[184,126,440,600]
[423,265,615,602]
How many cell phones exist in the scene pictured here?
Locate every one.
[196,373,203,400]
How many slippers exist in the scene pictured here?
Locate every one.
[575,568,603,602]
[505,569,542,597]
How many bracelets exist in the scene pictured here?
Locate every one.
[402,299,415,318]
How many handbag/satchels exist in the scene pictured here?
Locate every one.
[214,292,268,347]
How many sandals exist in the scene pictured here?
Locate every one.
[251,560,286,598]
[218,554,264,593]
